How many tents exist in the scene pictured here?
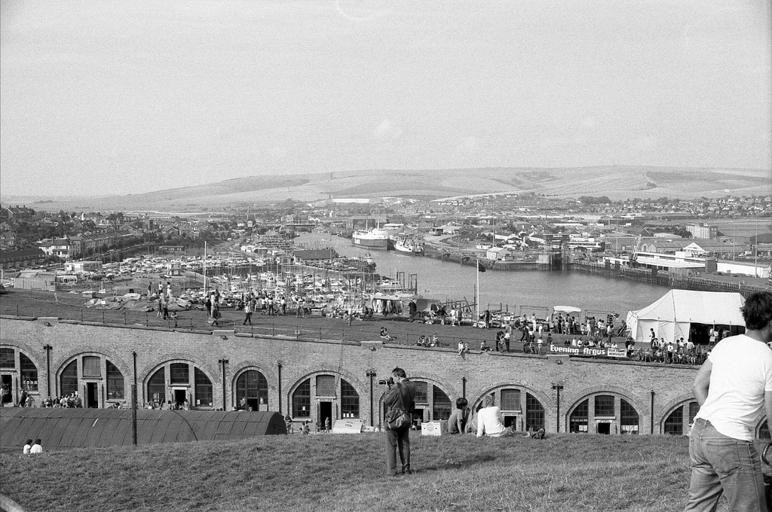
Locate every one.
[624,286,748,344]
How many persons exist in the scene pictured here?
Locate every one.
[30,438,45,453]
[458,340,470,359]
[481,310,731,365]
[446,396,477,435]
[0,383,255,411]
[408,299,462,327]
[146,276,320,328]
[680,290,771,512]
[381,365,415,475]
[380,327,393,342]
[22,438,33,454]
[474,392,515,438]
[284,413,332,434]
[417,335,439,347]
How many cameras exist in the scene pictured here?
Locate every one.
[379,377,394,385]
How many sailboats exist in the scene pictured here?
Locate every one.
[183,256,413,304]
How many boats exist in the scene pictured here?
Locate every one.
[394,234,423,253]
[351,219,394,251]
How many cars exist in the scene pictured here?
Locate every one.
[103,252,224,277]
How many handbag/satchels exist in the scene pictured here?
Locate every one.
[383,403,410,430]
